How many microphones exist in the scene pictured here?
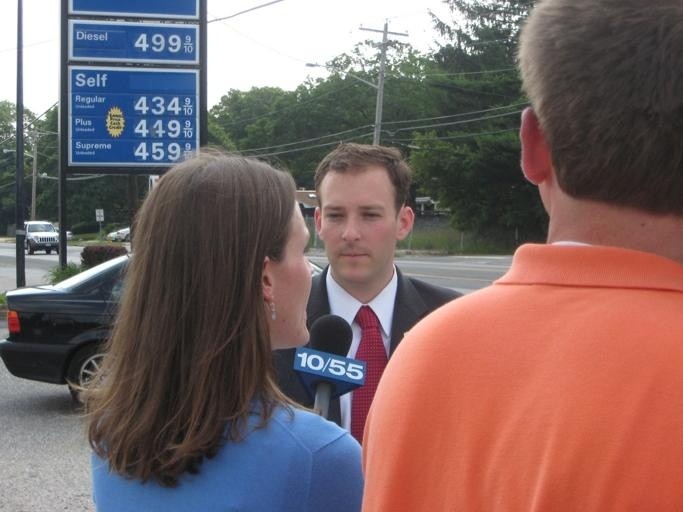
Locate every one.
[308,314,354,420]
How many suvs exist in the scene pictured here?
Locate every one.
[17,216,62,261]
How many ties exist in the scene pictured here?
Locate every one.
[351,306,389,446]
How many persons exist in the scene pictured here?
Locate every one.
[356,0,682,511]
[266,139,465,446]
[72,147,364,511]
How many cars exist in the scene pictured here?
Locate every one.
[105,225,134,245]
[0,251,328,407]
[53,225,73,241]
